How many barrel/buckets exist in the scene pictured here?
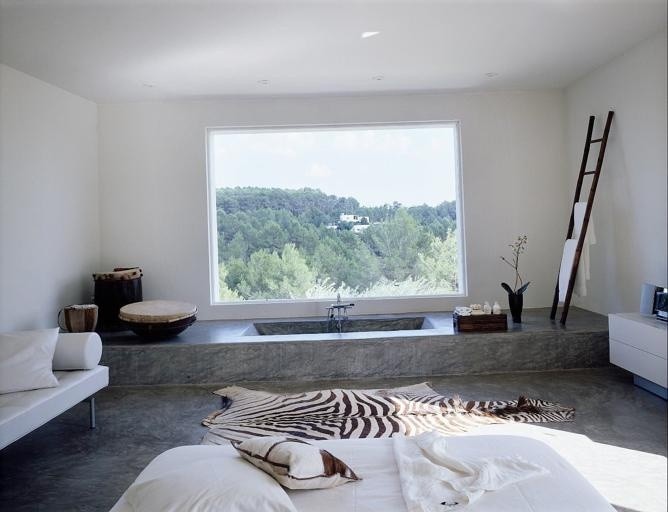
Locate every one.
[93,262,144,333]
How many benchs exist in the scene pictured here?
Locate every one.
[1,331,109,450]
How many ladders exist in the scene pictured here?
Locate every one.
[550,110,614,327]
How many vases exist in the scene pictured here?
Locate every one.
[501,282,530,324]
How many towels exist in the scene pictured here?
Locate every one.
[395,431,549,510]
[557,202,597,304]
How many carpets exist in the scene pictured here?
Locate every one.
[200,380,577,445]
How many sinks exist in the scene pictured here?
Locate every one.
[234,312,438,341]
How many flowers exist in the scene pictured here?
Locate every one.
[500,234,527,291]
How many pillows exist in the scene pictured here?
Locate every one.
[1,326,60,393]
[127,435,362,511]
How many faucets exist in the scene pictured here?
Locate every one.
[324,294,356,319]
